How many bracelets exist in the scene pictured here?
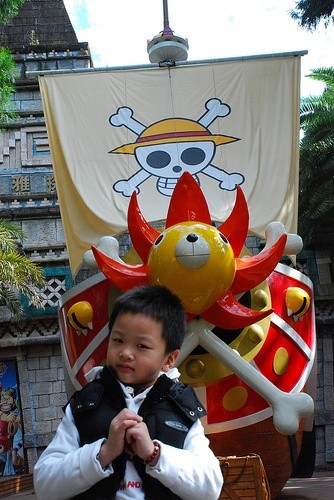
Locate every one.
[144,439,160,466]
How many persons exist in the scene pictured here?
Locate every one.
[27,283,225,500]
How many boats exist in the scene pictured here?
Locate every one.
[34,0,319,500]
